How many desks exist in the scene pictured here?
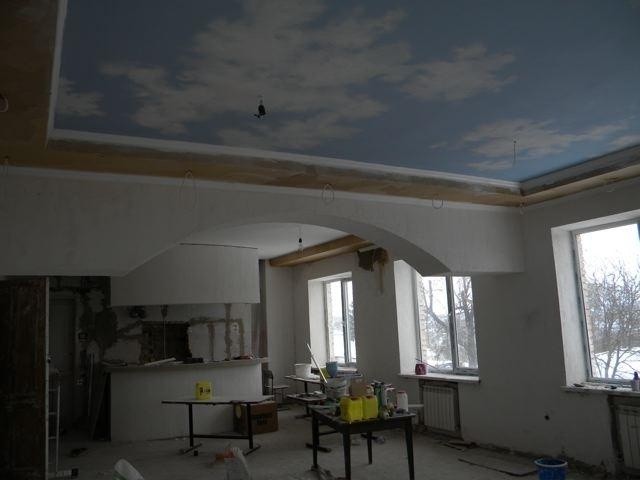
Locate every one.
[284,373,337,424]
[309,405,416,480]
[161,394,274,456]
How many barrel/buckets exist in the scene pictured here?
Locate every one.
[294,363,312,378]
[324,378,346,401]
[534,457,568,480]
